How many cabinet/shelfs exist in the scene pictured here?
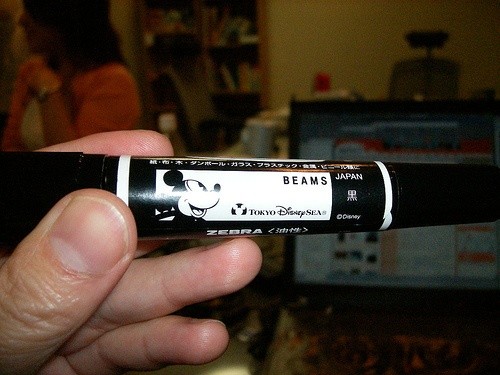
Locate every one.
[130,0,271,152]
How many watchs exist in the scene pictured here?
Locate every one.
[33,84,60,101]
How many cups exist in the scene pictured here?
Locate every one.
[247,120,275,157]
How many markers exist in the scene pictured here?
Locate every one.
[0,150,500,256]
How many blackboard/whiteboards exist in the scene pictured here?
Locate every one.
[257,0,500,113]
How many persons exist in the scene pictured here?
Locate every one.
[0,130,262,375]
[1,0,142,153]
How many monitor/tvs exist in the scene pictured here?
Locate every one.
[286,97,500,314]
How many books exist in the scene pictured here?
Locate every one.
[200,6,257,46]
[144,8,182,46]
[218,62,256,94]
